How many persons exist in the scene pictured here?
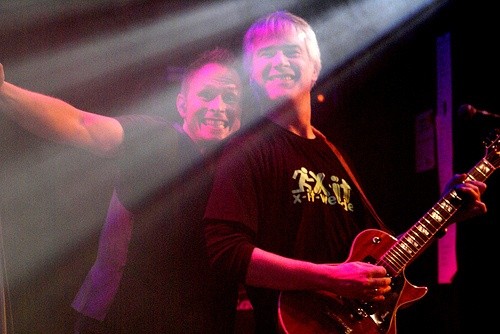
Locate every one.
[0,47,261,334]
[205,12,487,334]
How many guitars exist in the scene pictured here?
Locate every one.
[275,135,500,334]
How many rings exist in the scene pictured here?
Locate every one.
[374,288,377,294]
